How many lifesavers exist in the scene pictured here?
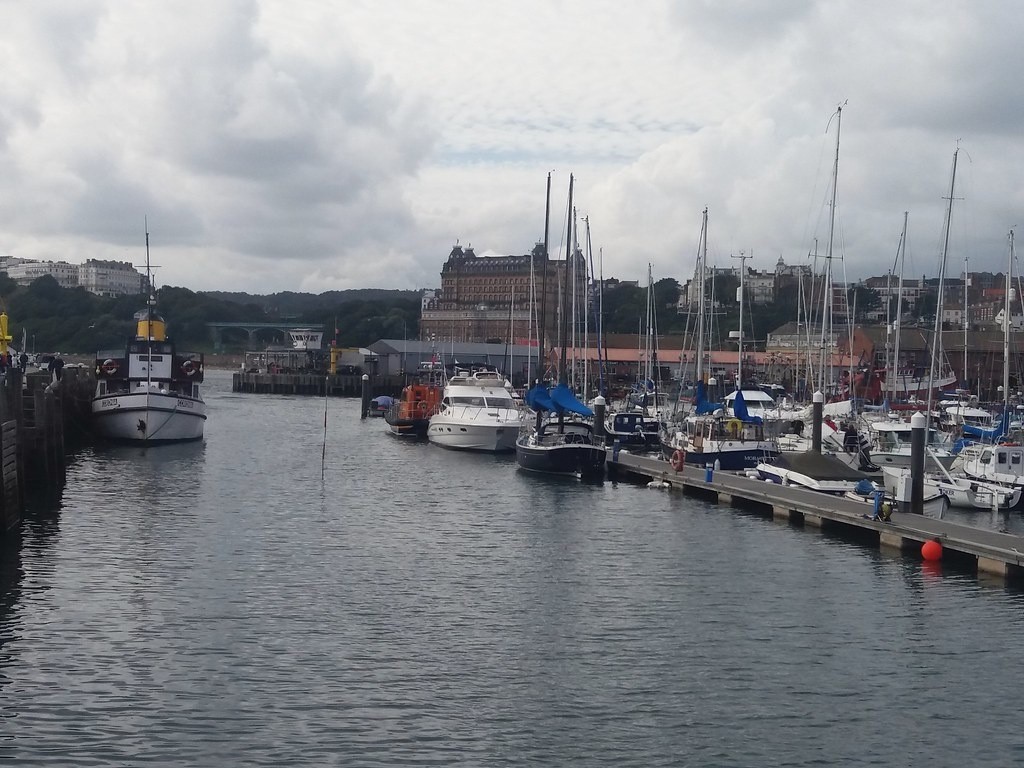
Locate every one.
[671,450,683,471]
[102,359,118,374]
[417,400,432,414]
[1000,443,1022,446]
[181,360,197,376]
[726,418,742,434]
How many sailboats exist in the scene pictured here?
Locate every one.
[548,101,1024,511]
[515,169,607,481]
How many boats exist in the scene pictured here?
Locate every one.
[427,369,537,455]
[382,369,443,438]
[91,214,208,448]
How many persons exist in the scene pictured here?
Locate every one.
[964,398,981,423]
[844,425,857,452]
[842,368,865,402]
[1,350,64,381]
